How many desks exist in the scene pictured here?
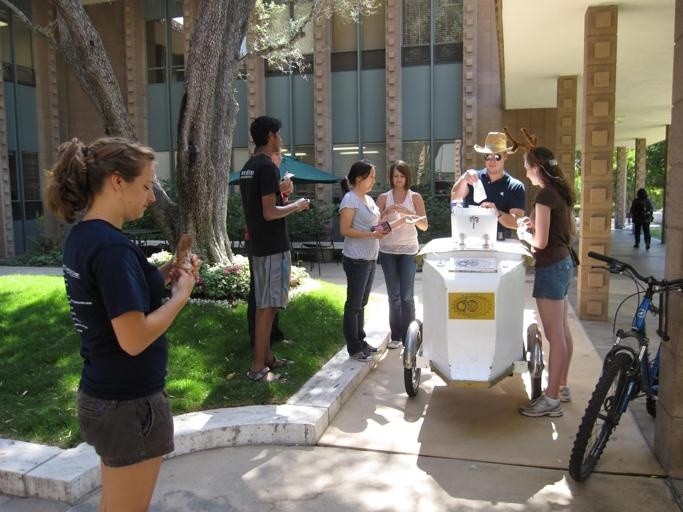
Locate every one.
[125,230,163,246]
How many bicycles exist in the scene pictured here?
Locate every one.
[562,249,682,489]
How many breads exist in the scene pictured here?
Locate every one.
[175,233,194,279]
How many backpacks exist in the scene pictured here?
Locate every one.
[637,198,651,218]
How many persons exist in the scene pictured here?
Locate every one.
[43,135,203,512]
[239,115,311,384]
[515,146,577,420]
[630,188,654,250]
[375,159,429,349]
[449,131,525,239]
[243,145,296,351]
[339,159,423,362]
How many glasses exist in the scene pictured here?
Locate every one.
[484,153,505,162]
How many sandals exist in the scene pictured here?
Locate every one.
[245,326,299,386]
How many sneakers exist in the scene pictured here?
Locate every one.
[516,397,564,419]
[346,335,404,362]
[632,243,652,252]
[540,384,570,403]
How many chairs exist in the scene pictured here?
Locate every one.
[291,228,338,275]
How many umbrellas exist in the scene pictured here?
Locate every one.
[228,152,341,185]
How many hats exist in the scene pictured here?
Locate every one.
[472,129,520,155]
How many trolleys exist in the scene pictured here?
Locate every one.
[399,202,543,410]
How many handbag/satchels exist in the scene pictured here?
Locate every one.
[369,221,391,236]
[567,246,581,268]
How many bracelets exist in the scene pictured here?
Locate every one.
[498,210,502,217]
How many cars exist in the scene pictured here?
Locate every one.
[651,206,663,225]
